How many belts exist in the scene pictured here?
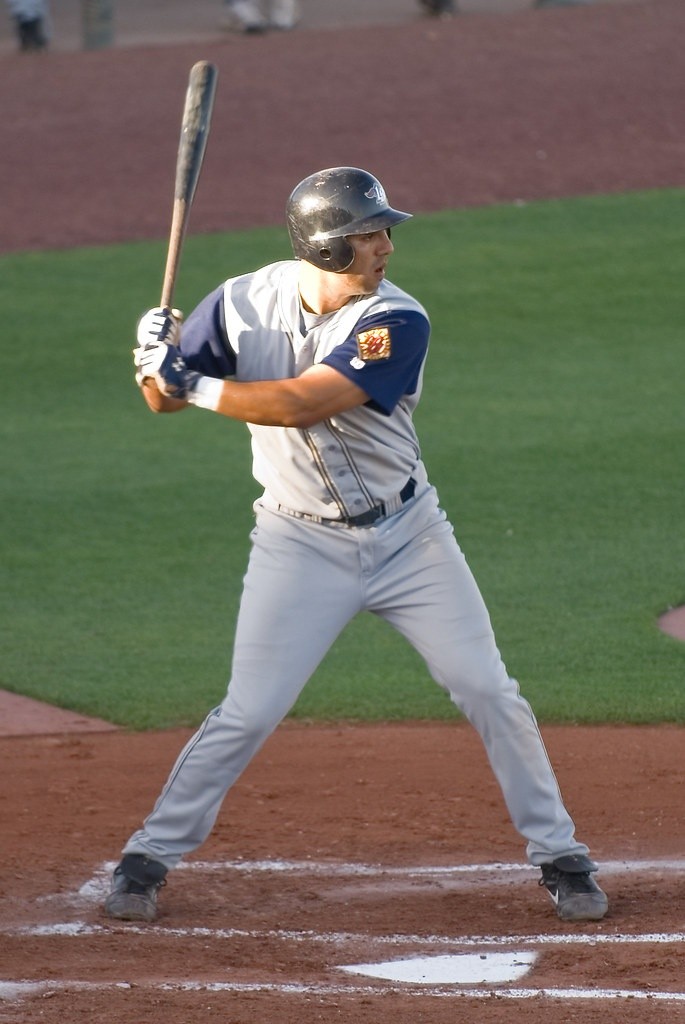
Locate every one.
[321,477,417,527]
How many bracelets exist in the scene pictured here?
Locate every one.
[178,370,225,411]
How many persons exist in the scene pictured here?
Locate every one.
[6,0,458,56]
[106,168,609,923]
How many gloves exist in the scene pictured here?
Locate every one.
[132,341,224,412]
[134,307,183,390]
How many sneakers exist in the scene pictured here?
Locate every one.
[537,855,608,919]
[104,854,169,922]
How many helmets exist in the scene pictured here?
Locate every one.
[285,166,414,273]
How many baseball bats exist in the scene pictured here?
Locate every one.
[155,58,221,344]
